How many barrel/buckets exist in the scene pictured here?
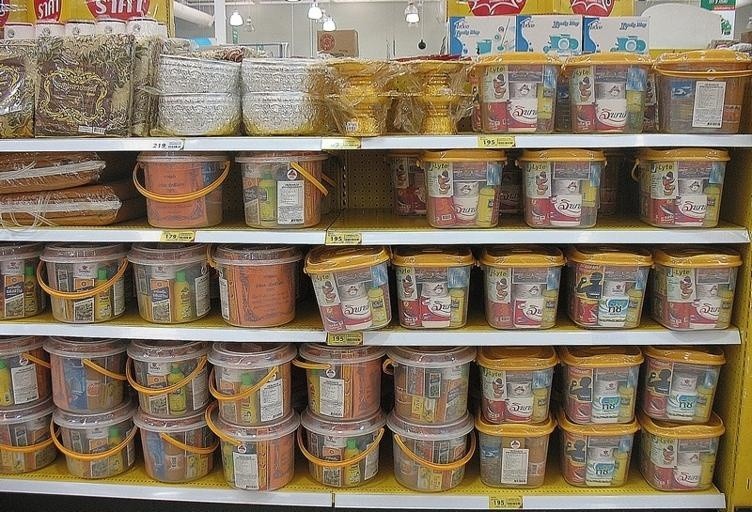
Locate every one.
[46,404,139,480]
[1,336,62,478]
[132,152,337,228]
[199,398,295,493]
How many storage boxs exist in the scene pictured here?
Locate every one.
[476,400,557,489]
[236,151,336,231]
[639,47,751,133]
[566,245,654,330]
[477,247,567,332]
[633,151,732,229]
[558,51,654,132]
[317,27,361,59]
[552,398,638,490]
[1,336,52,409]
[470,45,562,134]
[520,147,602,229]
[133,153,235,228]
[301,244,393,332]
[439,9,653,56]
[477,345,560,423]
[419,150,509,230]
[632,403,727,491]
[389,247,476,328]
[49,386,135,483]
[642,345,726,425]
[206,401,303,490]
[386,150,634,217]
[557,345,645,424]
[2,398,60,474]
[652,246,742,332]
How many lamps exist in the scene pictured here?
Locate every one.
[402,1,424,24]
[306,1,338,33]
[228,3,257,33]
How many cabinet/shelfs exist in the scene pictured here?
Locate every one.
[1,125,752,510]
